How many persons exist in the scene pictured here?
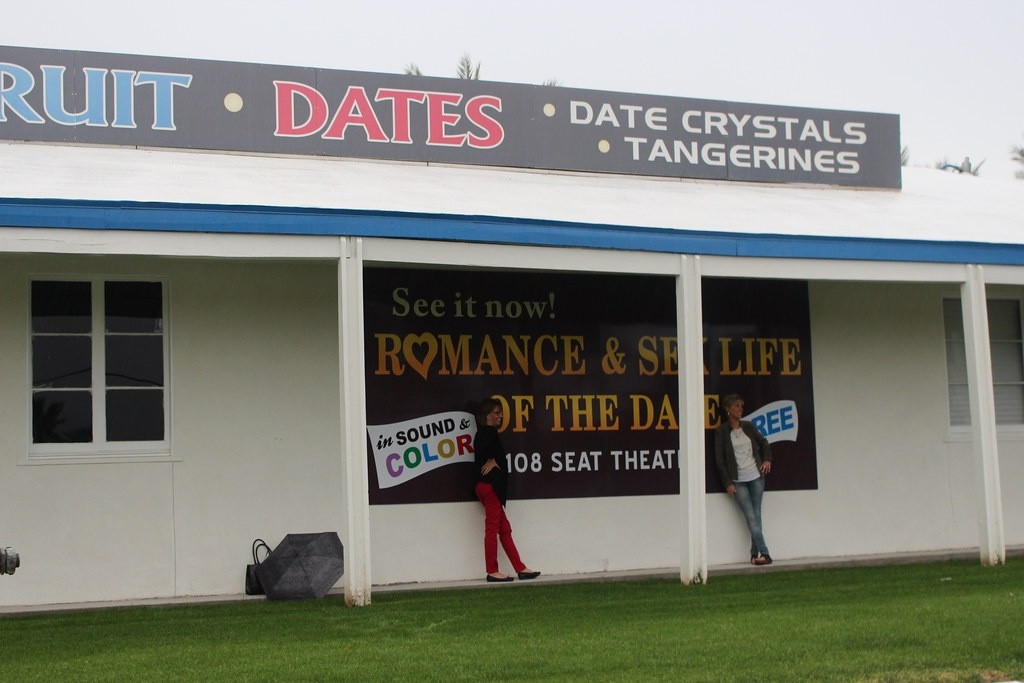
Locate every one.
[714,395,774,566]
[472,397,541,581]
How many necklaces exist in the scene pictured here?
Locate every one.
[733,428,743,438]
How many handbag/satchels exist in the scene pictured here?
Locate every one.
[245,539,272,595]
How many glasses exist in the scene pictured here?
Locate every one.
[492,411,500,416]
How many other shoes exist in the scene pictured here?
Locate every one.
[518,571,541,580]
[755,554,772,564]
[487,574,514,582]
[750,556,758,564]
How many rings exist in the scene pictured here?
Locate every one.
[763,466,766,468]
[769,466,771,468]
